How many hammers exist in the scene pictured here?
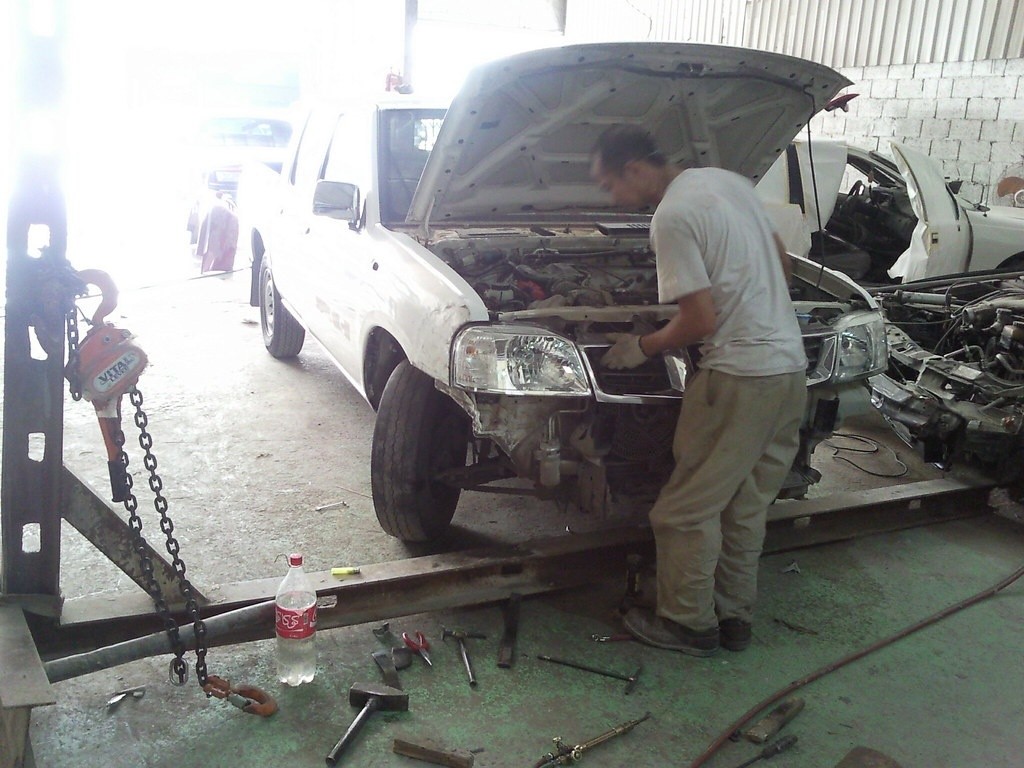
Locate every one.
[440,626,488,687]
[325,681,409,765]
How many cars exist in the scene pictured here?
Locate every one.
[755,138,1024,284]
[233,40,888,544]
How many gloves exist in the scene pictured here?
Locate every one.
[604,333,647,370]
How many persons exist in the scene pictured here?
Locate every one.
[589,124,808,657]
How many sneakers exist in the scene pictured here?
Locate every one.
[623,607,720,657]
[720,617,751,651]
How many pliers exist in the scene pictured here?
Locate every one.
[402,630,432,666]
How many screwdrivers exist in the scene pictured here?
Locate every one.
[735,734,799,768]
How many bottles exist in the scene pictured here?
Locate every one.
[274,554,318,687]
